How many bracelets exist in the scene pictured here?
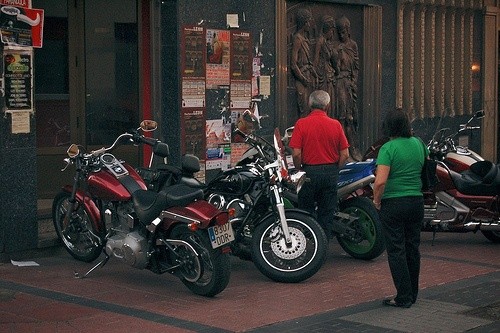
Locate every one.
[373,200,381,206]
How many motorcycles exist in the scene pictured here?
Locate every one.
[52,120,231,296]
[235,110,500,259]
[135,128,328,283]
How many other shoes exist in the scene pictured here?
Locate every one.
[383,295,415,308]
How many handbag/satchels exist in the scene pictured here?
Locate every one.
[412,134,438,191]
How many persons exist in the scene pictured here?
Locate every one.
[290,6,361,162]
[288,90,350,250]
[373,109,426,308]
